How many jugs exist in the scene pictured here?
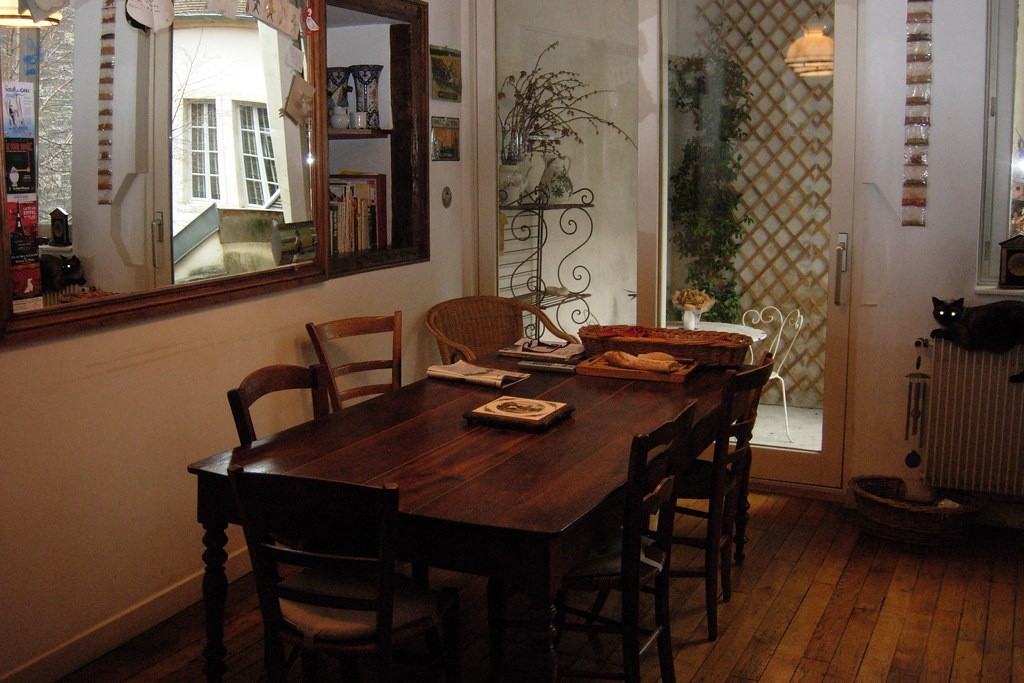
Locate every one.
[330,106,350,129]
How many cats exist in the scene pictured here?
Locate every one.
[930,296,1024,352]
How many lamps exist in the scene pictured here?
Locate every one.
[784,23,833,78]
[0,0,64,27]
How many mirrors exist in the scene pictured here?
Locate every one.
[0,0,334,353]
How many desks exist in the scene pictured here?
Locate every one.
[187,349,768,683]
[662,322,766,365]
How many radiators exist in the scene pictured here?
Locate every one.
[921,338,1024,497]
[42,282,82,307]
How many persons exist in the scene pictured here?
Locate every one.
[292,230,304,263]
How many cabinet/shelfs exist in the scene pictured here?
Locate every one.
[498,185,596,341]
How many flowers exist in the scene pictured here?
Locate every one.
[491,40,639,166]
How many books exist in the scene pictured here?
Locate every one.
[494,337,589,375]
[329,170,387,261]
[426,359,532,390]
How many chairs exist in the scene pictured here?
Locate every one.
[305,311,430,583]
[226,364,333,574]
[588,352,773,639]
[742,308,807,418]
[424,294,580,365]
[482,400,704,683]
[226,464,464,683]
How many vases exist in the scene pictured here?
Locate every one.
[497,159,527,205]
[540,151,573,203]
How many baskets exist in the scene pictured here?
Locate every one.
[849,474,979,549]
[579,325,752,367]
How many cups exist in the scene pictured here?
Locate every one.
[350,111,367,129]
